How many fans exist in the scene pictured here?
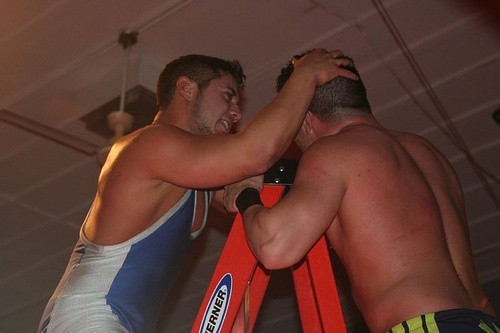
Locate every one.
[0,108,136,167]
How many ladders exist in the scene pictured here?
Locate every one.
[191,158,347,333]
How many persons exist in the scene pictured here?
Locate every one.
[226,49,500,333]
[36,49,359,333]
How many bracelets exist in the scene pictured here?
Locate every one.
[235,187,264,215]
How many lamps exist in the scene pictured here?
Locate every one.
[79,84,158,141]
